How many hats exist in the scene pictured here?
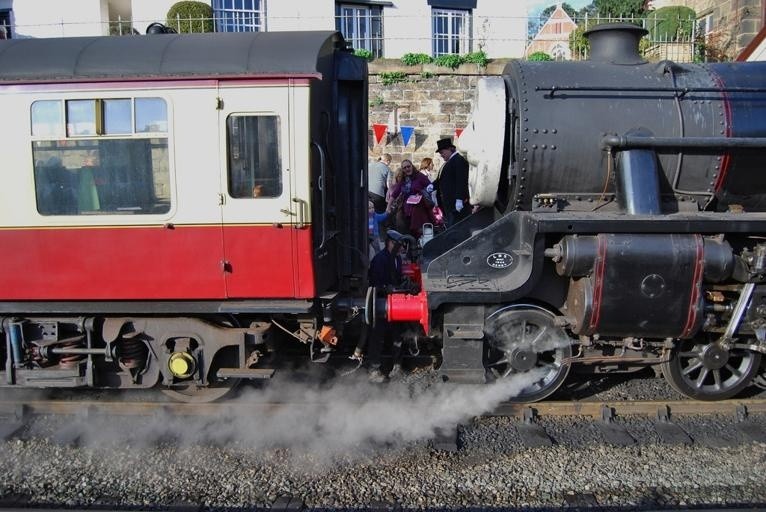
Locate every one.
[435,138,456,152]
[386,229,407,246]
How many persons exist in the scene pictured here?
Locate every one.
[369,230,409,382]
[368,138,469,255]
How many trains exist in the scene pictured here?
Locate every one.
[0,23,766,400]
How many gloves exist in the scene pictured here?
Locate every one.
[426,184,434,193]
[454,199,465,213]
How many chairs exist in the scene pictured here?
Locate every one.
[34,156,150,214]
